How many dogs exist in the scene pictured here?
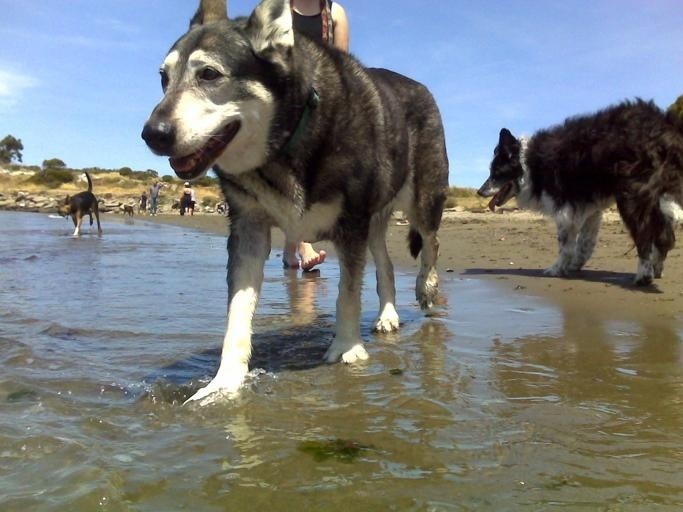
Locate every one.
[55,171,103,236]
[118,202,135,218]
[141,0,449,407]
[477,98,683,286]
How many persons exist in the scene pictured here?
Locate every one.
[282,1,349,270]
[148,182,158,217]
[182,182,191,214]
[188,184,195,215]
[137,191,147,214]
